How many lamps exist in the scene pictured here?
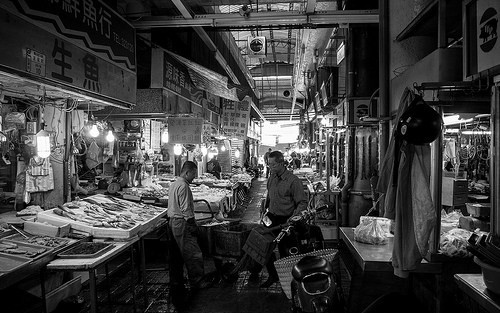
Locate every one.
[36,95,51,159]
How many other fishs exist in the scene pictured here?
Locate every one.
[53,194,166,229]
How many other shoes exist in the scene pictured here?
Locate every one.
[261,275,279,287]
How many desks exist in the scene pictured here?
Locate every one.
[454,273,500,312]
[196,181,241,212]
[41,218,169,313]
[307,183,341,224]
[338,225,443,313]
[124,192,230,218]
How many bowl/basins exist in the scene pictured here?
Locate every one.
[473,255,500,295]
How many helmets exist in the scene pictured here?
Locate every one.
[397,103,442,145]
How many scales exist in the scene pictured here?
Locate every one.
[459,203,490,231]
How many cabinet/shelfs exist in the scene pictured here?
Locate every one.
[442,176,468,207]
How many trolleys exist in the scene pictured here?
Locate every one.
[189,198,269,285]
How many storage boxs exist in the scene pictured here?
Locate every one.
[213,222,261,257]
[197,217,242,247]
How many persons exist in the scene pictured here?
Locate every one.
[264,147,273,178]
[260,150,309,289]
[166,160,210,294]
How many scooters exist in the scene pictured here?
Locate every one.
[274,205,340,313]
